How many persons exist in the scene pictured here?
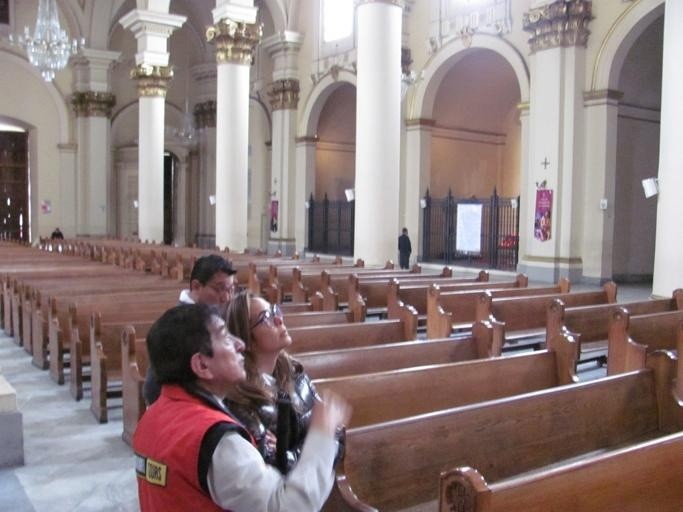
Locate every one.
[534,207,552,241]
[50,227,64,241]
[130,303,350,511]
[398,228,412,268]
[224,290,344,477]
[141,254,237,408]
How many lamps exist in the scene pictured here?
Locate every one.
[8,0,85,82]
[345,189,355,202]
[208,195,216,206]
[642,177,659,199]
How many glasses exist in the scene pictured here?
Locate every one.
[203,281,234,297]
[250,304,284,331]
